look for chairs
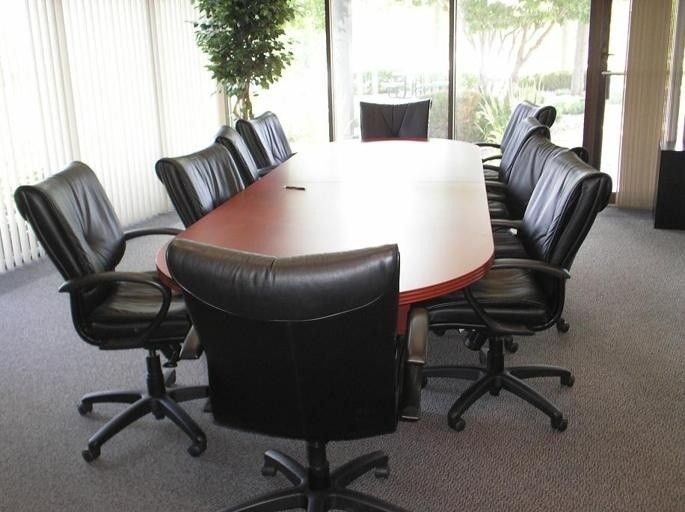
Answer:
[358,99,431,142]
[424,98,590,352]
[13,160,214,462]
[155,144,244,228]
[213,125,279,189]
[13,96,613,512]
[166,235,409,512]
[235,110,299,170]
[423,151,612,433]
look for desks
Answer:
[651,144,685,230]
[154,138,495,389]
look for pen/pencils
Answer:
[286,186,305,190]
